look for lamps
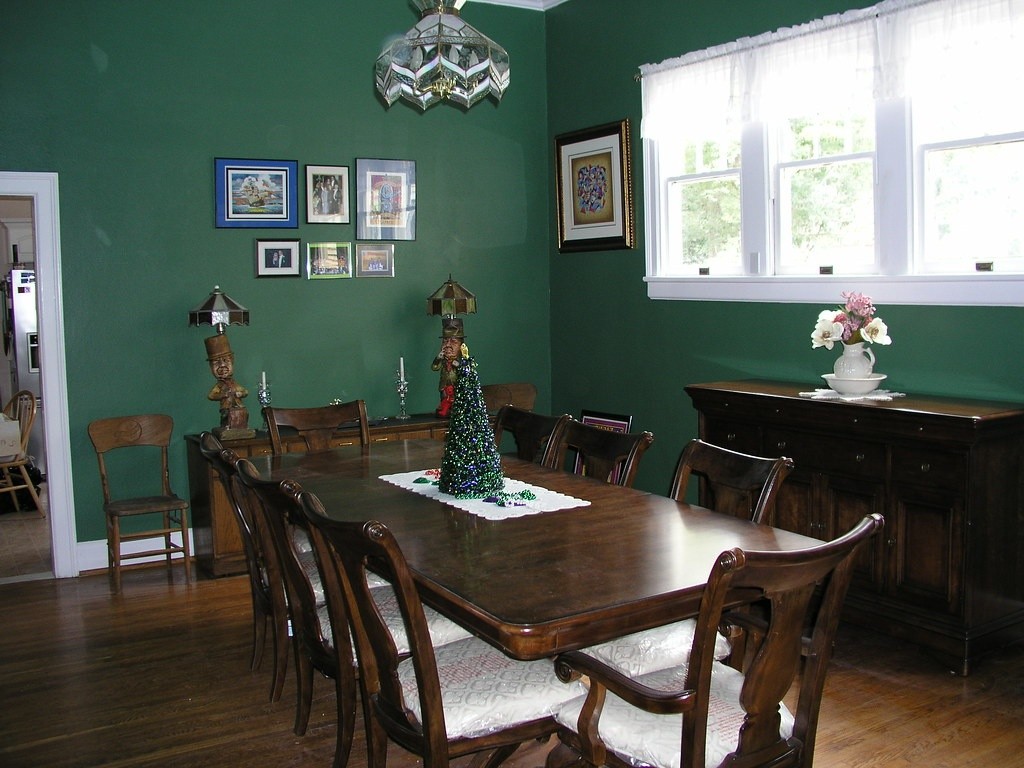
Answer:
[427,272,478,416]
[372,0,511,111]
[187,285,258,441]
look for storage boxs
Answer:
[0,418,22,457]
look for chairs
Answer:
[545,416,655,487]
[493,404,568,466]
[197,427,392,705]
[545,511,886,768]
[262,395,371,552]
[87,414,192,591]
[0,390,47,518]
[278,477,589,768]
[481,383,537,416]
[233,458,474,768]
[576,437,795,679]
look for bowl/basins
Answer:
[820,373,888,397]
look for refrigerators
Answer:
[10,270,46,474]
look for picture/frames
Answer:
[575,410,633,484]
[356,244,396,278]
[554,119,635,253]
[355,157,417,241]
[307,242,353,280]
[305,164,350,225]
[255,238,302,279]
[214,158,299,229]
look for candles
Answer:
[262,371,267,398]
[399,357,404,383]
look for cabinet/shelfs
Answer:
[181,411,497,582]
[682,380,1024,678]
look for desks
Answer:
[247,440,828,768]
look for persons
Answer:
[432,318,467,408]
[204,334,248,427]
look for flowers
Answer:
[808,291,892,349]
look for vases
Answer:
[833,339,875,378]
